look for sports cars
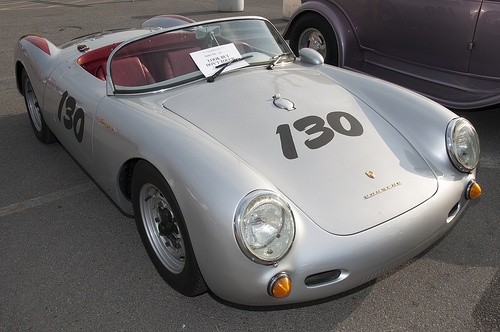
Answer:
[13,15,482,309]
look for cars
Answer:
[281,0,500,111]
